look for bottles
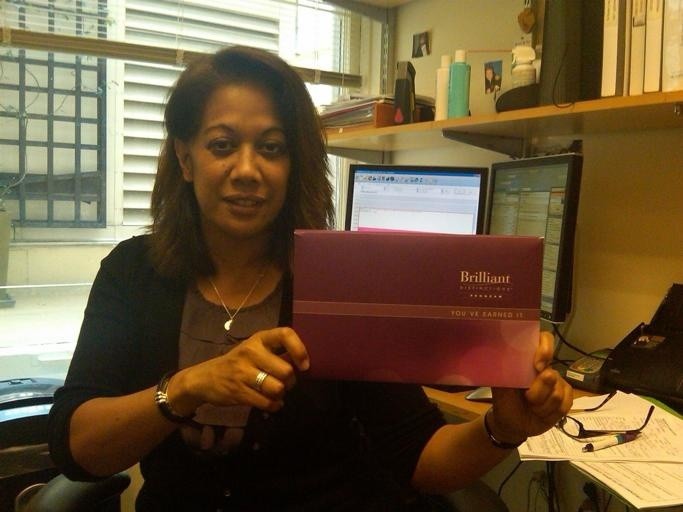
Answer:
[434,54,451,120]
[447,49,470,118]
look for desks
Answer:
[420,376,682,511]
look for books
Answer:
[534,1,683,107]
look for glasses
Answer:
[550,391,655,440]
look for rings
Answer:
[253,371,267,391]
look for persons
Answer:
[414,33,429,56]
[46,46,573,512]
[484,62,499,93]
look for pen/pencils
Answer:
[582,432,637,453]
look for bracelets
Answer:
[483,408,523,448]
[154,369,186,425]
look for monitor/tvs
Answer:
[483,151,584,324]
[344,164,490,235]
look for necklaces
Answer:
[208,265,263,332]
